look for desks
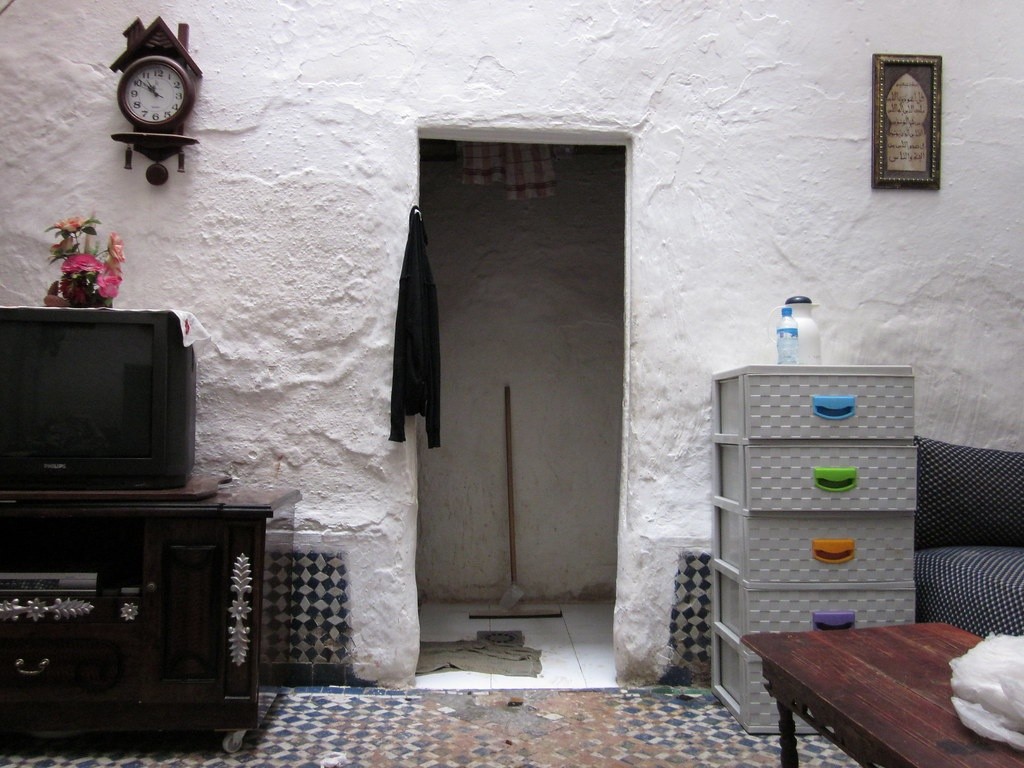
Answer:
[742,623,1024,768]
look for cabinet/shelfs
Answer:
[711,366,916,735]
[0,488,301,750]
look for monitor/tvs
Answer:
[0,307,197,490]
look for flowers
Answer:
[44,216,125,307]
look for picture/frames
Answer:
[872,54,942,190]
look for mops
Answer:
[468,384,564,619]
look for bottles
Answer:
[777,307,801,365]
[786,294,822,368]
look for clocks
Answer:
[111,16,203,187]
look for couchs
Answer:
[916,437,1024,639]
